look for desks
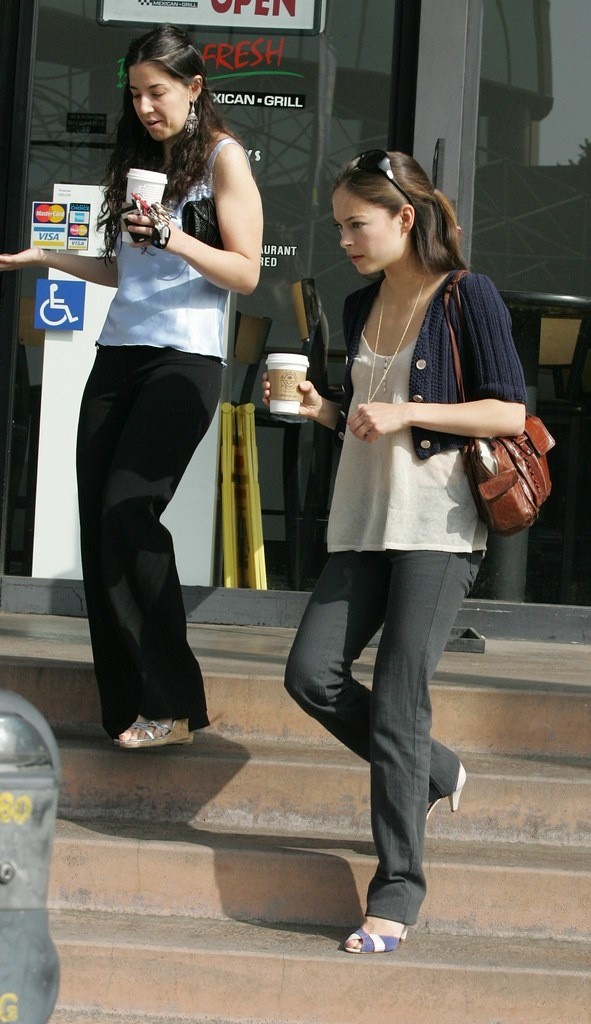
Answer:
[483,289,591,604]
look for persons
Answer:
[261,151,527,954]
[0,23,265,747]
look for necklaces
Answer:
[368,274,426,403]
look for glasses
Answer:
[354,149,414,208]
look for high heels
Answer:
[344,912,409,955]
[428,759,468,820]
[112,712,192,750]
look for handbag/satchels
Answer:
[441,268,557,535]
[182,196,223,249]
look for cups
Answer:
[266,353,309,415]
[122,168,168,243]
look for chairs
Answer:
[5,302,45,577]
[233,277,351,588]
[527,316,591,604]
